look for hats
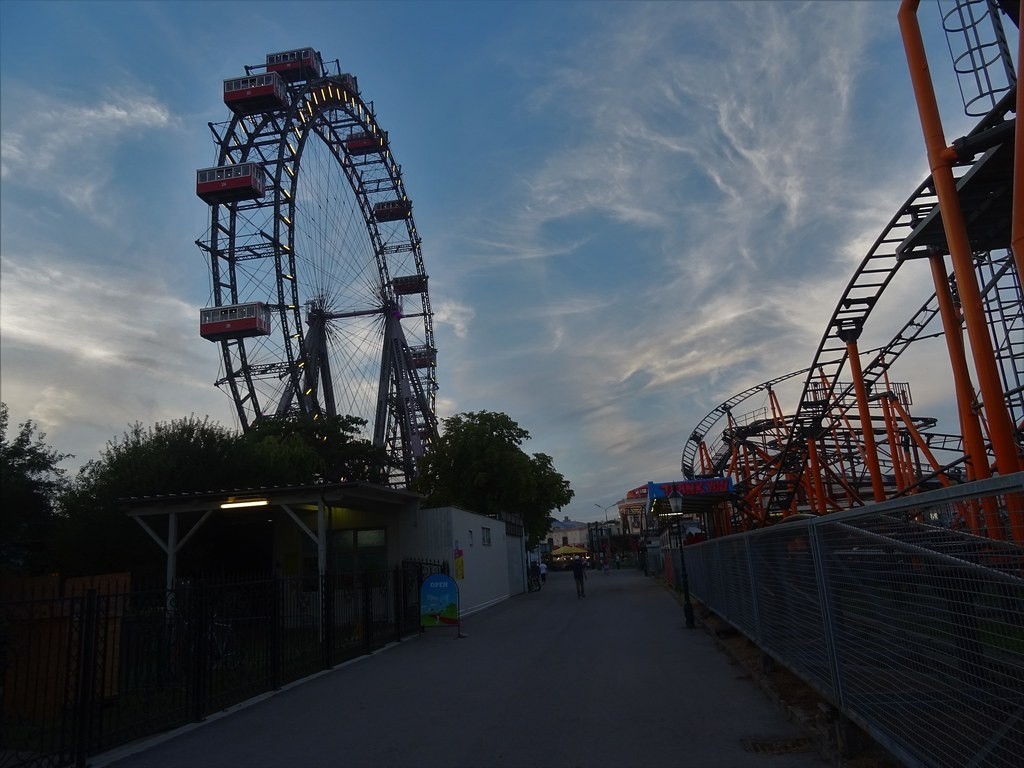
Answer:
[575,555,580,561]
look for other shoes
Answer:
[542,582,544,585]
[577,595,580,598]
[581,594,585,597]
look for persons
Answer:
[599,552,620,576]
[556,553,591,562]
[574,555,585,597]
[529,560,542,592]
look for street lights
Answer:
[667,480,695,629]
[595,502,618,521]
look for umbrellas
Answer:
[551,545,588,555]
